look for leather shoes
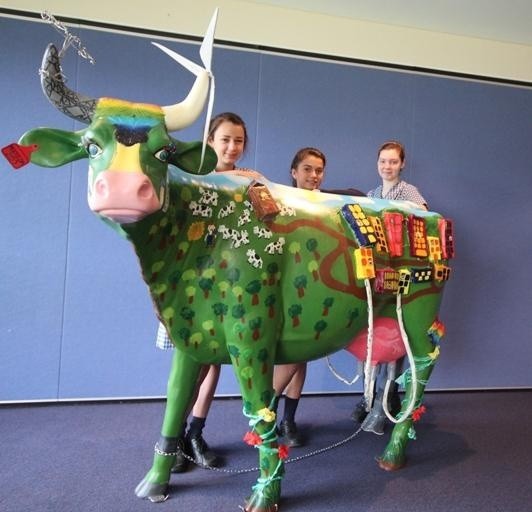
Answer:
[190,436,218,469]
[389,392,401,417]
[280,420,302,448]
[350,399,370,424]
[170,440,188,473]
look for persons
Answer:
[156,112,268,472]
[271,147,366,448]
[349,141,427,421]
